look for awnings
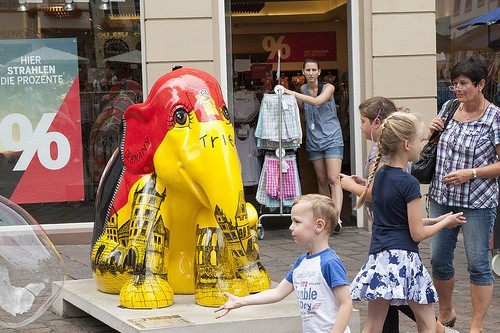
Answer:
[456,8,500,30]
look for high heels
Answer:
[440,310,456,327]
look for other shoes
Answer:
[333,220,342,233]
[444,325,460,333]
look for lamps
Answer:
[64,0,89,11]
[17,0,43,12]
[98,0,125,10]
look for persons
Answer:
[93,73,136,102]
[338,96,467,333]
[429,57,500,333]
[282,59,344,233]
[214,193,353,333]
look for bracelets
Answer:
[472,168,477,179]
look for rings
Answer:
[432,123,435,126]
[453,181,455,184]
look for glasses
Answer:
[449,82,476,91]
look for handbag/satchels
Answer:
[410,98,462,184]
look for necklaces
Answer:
[311,105,316,130]
[460,97,485,120]
